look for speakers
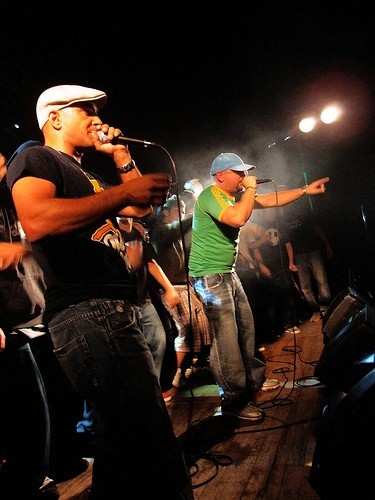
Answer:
[319,286,369,339]
[313,303,374,388]
[316,352,375,436]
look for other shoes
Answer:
[162,393,172,402]
[172,371,182,388]
[286,326,300,333]
[185,358,200,378]
[309,312,321,323]
[221,403,264,421]
[259,379,280,390]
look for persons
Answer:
[115,178,213,402]
[6,85,195,500]
[188,153,330,421]
[235,185,330,352]
[0,141,94,500]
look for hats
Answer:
[36,85,107,129]
[210,152,255,176]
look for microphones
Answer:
[95,130,155,146]
[237,177,270,191]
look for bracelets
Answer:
[302,184,311,197]
[115,159,135,173]
[245,186,257,194]
